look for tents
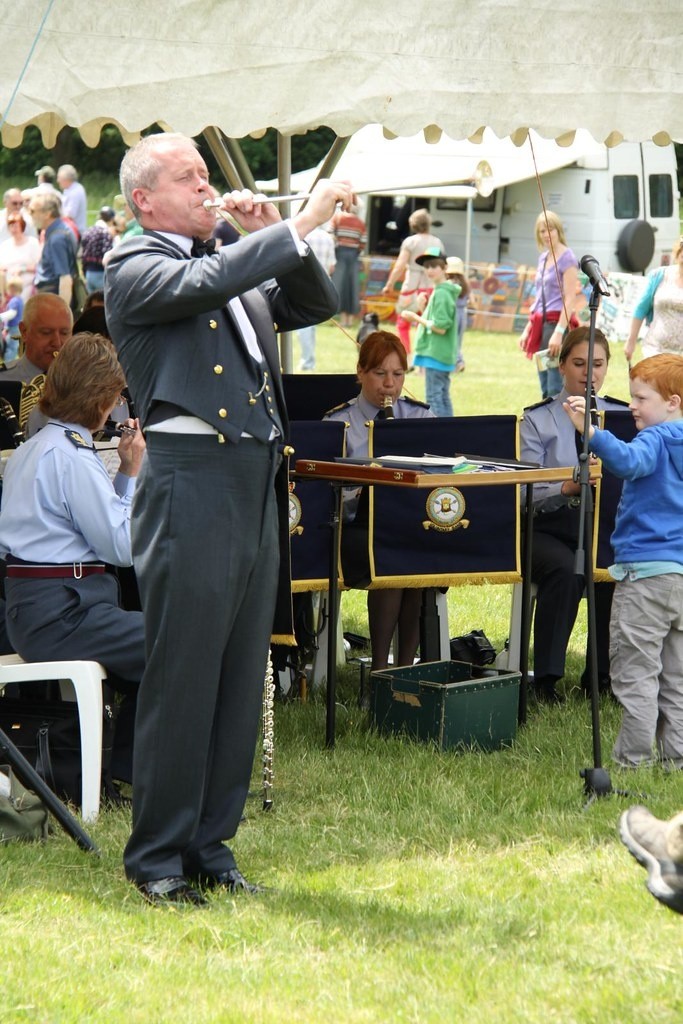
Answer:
[0,0,683,169]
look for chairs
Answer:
[0,336,650,853]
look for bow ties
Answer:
[191,234,220,259]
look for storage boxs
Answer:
[369,660,522,752]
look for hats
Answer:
[415,247,447,264]
[446,257,463,274]
[34,166,56,178]
[101,206,115,216]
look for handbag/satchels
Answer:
[450,629,497,666]
[72,277,90,321]
[0,764,56,842]
[534,345,565,371]
[0,693,119,804]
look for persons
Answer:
[625,238,683,366]
[562,353,683,773]
[400,247,462,416]
[0,332,146,807]
[382,209,444,374]
[323,331,437,697]
[288,192,367,372]
[0,164,153,451]
[518,325,633,701]
[445,257,469,372]
[103,132,357,912]
[621,806,683,916]
[519,209,579,402]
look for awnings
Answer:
[255,122,590,294]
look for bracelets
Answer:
[554,326,565,334]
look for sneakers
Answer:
[195,866,280,897]
[136,874,209,905]
[619,805,683,912]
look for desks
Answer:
[294,457,614,794]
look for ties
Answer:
[377,410,387,420]
[575,428,597,502]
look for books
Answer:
[334,450,548,473]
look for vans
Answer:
[255,124,679,275]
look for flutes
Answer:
[0,397,25,447]
[585,381,598,489]
[380,393,395,419]
[105,420,136,436]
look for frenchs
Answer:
[18,350,58,432]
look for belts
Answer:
[4,567,105,578]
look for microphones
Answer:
[580,255,611,297]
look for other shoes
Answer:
[457,361,464,371]
[535,683,563,702]
[575,690,604,702]
